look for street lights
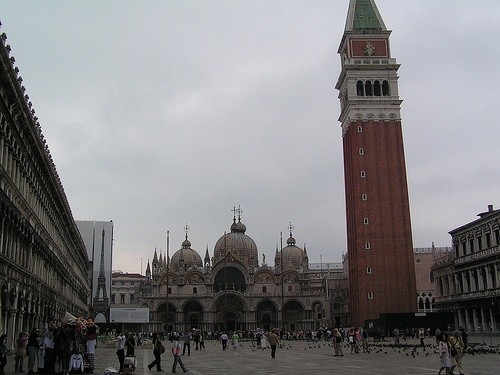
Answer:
[166,230,170,331]
[90,220,96,306]
[279,232,285,330]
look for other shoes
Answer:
[14,369,19,373]
[157,368,163,372]
[19,370,25,373]
[28,370,36,374]
[438,372,440,375]
[184,370,188,373]
[446,374,449,375]
[460,373,464,375]
[335,354,339,356]
[339,355,344,356]
[148,365,152,371]
[173,371,177,373]
[450,372,455,375]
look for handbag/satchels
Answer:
[154,342,163,357]
[335,333,342,343]
[349,333,354,343]
[25,344,30,356]
[451,350,458,357]
[171,342,181,354]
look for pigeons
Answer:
[247,342,293,351]
[205,340,244,350]
[340,340,351,348]
[468,343,497,356]
[304,341,334,351]
[358,343,433,358]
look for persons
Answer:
[0,317,474,375]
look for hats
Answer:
[31,328,40,333]
[86,319,94,325]
[127,332,134,338]
[454,329,459,335]
